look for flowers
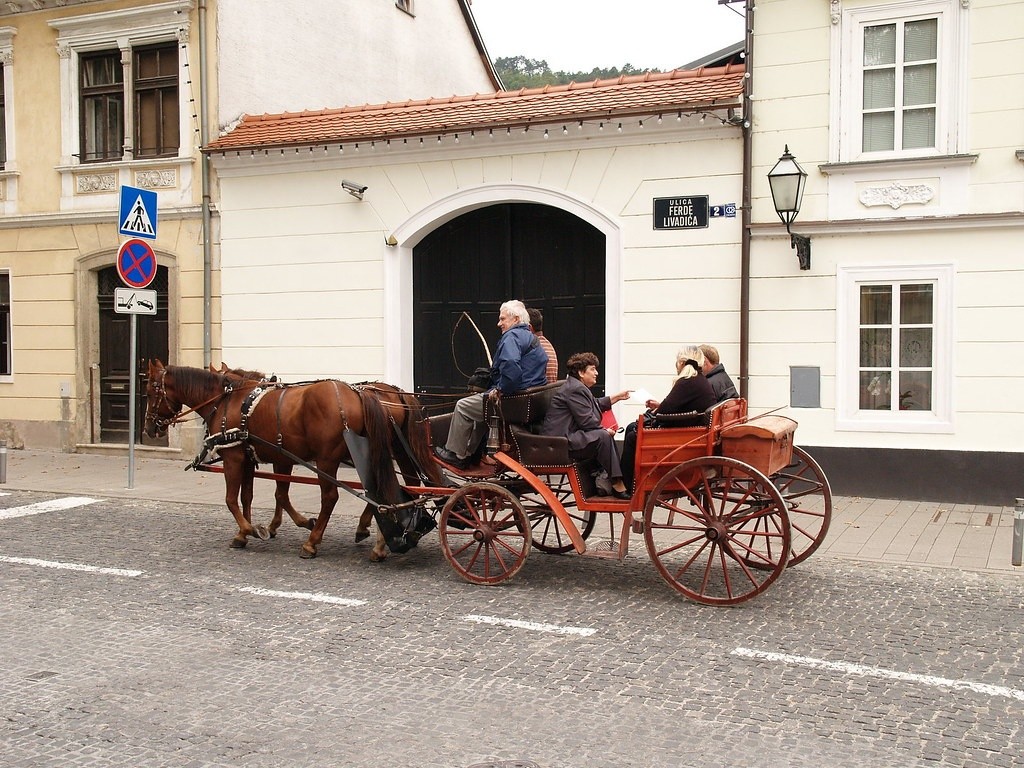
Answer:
[867,373,924,409]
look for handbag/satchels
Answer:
[634,409,661,434]
[591,468,613,496]
[466,368,494,395]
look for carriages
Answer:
[141,356,834,607]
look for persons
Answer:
[434,300,548,469]
[619,345,717,493]
[700,345,739,402]
[527,309,558,384]
[866,373,890,408]
[542,353,635,500]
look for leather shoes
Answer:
[470,454,480,466]
[612,485,632,500]
[436,447,471,470]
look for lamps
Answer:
[767,144,811,270]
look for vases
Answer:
[900,406,907,410]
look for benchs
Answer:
[635,397,747,490]
[482,379,593,475]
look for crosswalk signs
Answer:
[118,183,159,241]
